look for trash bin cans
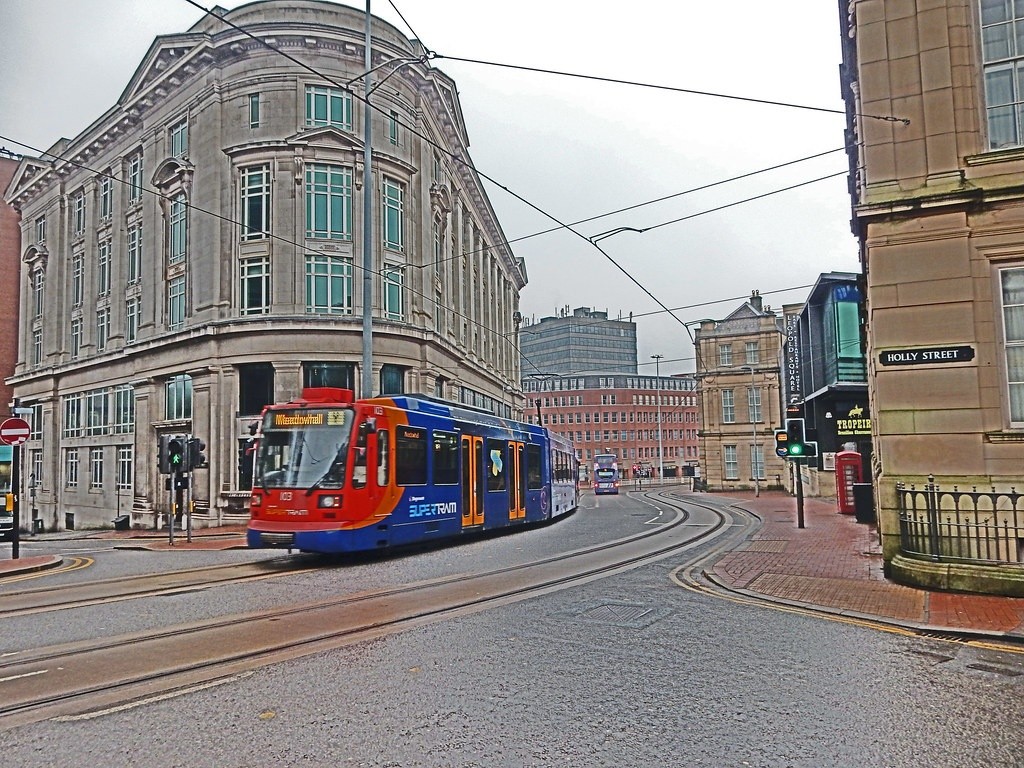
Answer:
[852,482,875,522]
[693,476,701,489]
[34,518,44,533]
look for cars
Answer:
[0,497,13,542]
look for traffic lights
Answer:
[786,417,819,458]
[190,438,206,466]
[166,435,188,471]
[5,494,13,511]
[158,431,170,475]
[238,441,252,476]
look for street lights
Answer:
[650,354,665,485]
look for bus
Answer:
[246,371,582,557]
[593,454,620,495]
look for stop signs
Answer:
[0,419,32,446]
